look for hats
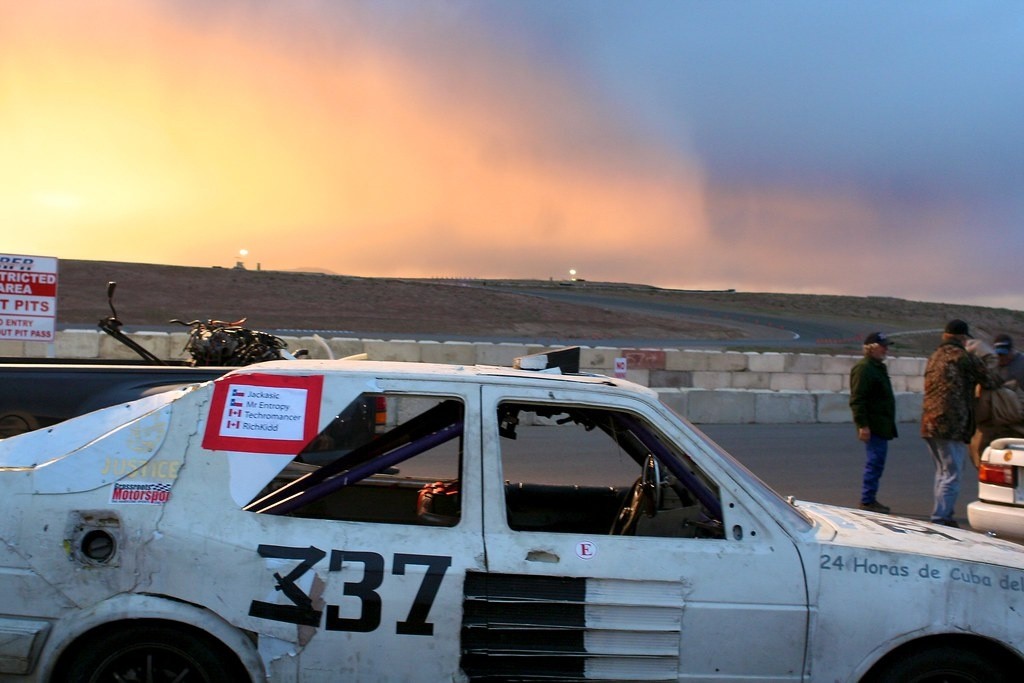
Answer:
[992,334,1011,354]
[945,319,975,340]
[864,332,895,346]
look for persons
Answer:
[921,319,1024,527]
[850,332,898,513]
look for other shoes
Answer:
[931,519,959,528]
[857,500,890,514]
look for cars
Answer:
[966,438,1024,542]
[1,347,1024,683]
